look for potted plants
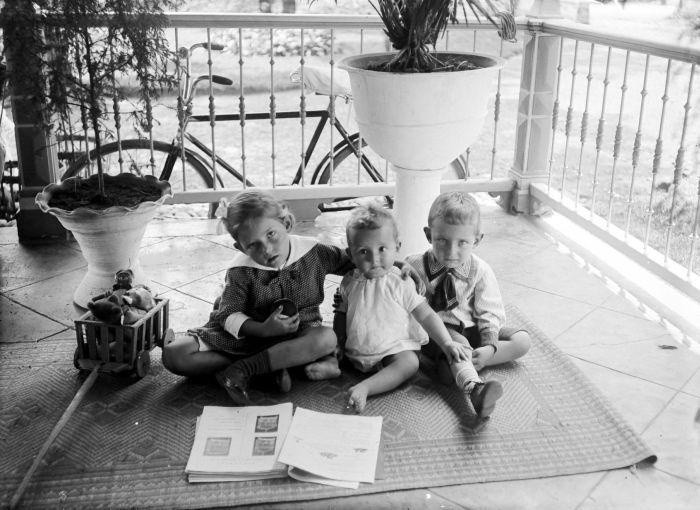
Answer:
[337,0,518,265]
[0,0,192,312]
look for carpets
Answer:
[0,297,656,509]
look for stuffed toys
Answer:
[86,270,156,326]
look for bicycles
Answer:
[56,39,474,217]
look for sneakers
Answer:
[470,380,503,418]
[215,362,291,406]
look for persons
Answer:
[304,201,473,413]
[162,189,427,405]
[331,190,531,417]
